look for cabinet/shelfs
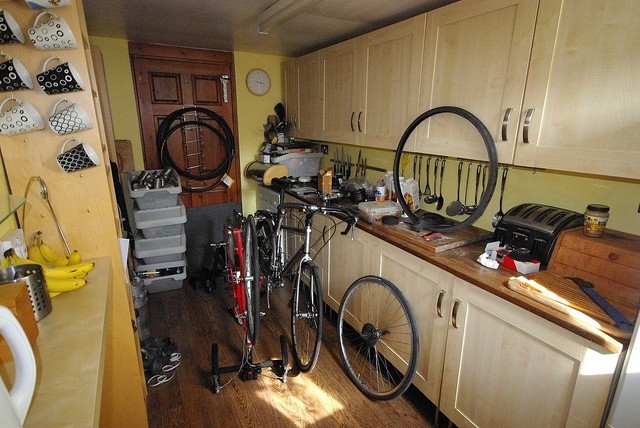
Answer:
[372,229,631,428]
[322,211,371,344]
[427,0,640,184]
[319,11,427,155]
[0,0,152,427]
[280,49,319,140]
[283,188,323,300]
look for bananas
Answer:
[1,247,94,299]
[28,230,81,266]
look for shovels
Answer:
[424,158,431,195]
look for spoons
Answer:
[424,158,440,203]
[491,168,508,228]
[445,162,463,216]
[465,164,481,214]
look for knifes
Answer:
[333,145,352,180]
[354,149,361,176]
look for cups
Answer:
[0,8,26,44]
[46,98,94,136]
[27,10,79,50]
[25,0,72,10]
[35,56,87,95]
[0,96,46,137]
[0,52,35,91]
[56,138,101,175]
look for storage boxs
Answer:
[269,150,323,176]
[127,167,182,209]
[133,253,188,294]
[132,232,186,264]
[132,196,188,238]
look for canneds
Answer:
[583,204,610,239]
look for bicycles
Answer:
[254,202,359,374]
[203,207,291,392]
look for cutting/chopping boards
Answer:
[371,208,494,253]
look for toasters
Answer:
[491,201,586,269]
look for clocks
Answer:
[246,69,271,96]
